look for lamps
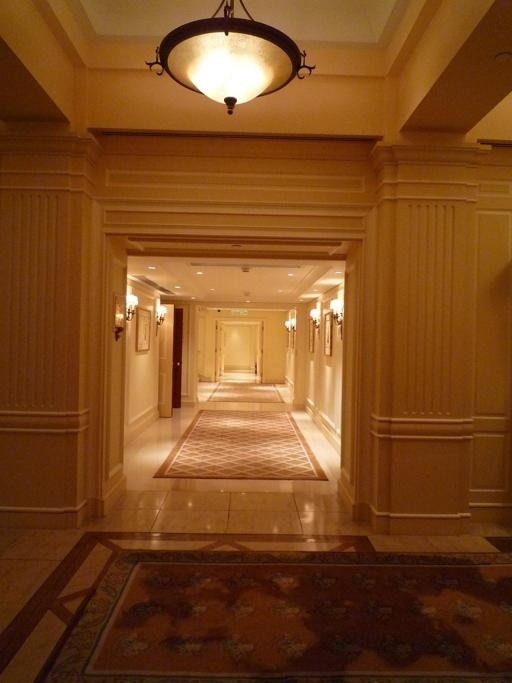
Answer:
[145,0,313,112]
[286,319,296,332]
[126,293,138,320]
[310,309,320,333]
[156,305,167,335]
[113,295,124,340]
[330,298,342,338]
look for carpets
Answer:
[207,382,284,403]
[30,549,511,682]
[152,408,329,481]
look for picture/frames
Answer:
[136,307,151,352]
[324,311,332,356]
[308,318,314,352]
